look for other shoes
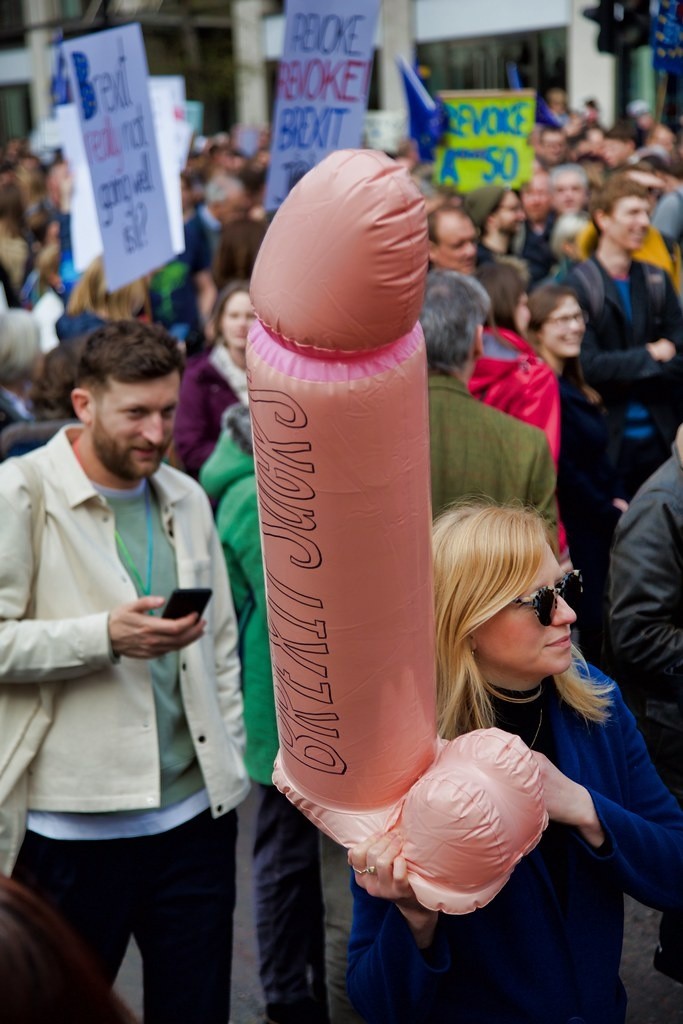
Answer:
[264,1002,332,1024]
[652,944,683,984]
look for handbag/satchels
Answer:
[0,456,59,878]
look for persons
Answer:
[2,85,682,671]
[345,494,683,1024]
[1,869,142,1023]
[199,402,330,1023]
[0,320,251,1024]
[416,268,559,562]
[600,422,683,811]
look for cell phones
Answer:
[161,588,213,623]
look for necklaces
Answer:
[530,707,543,751]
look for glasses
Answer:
[509,570,583,627]
[543,311,589,327]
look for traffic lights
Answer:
[582,0,613,57]
[615,2,651,53]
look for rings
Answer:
[352,865,366,874]
[365,864,376,875]
